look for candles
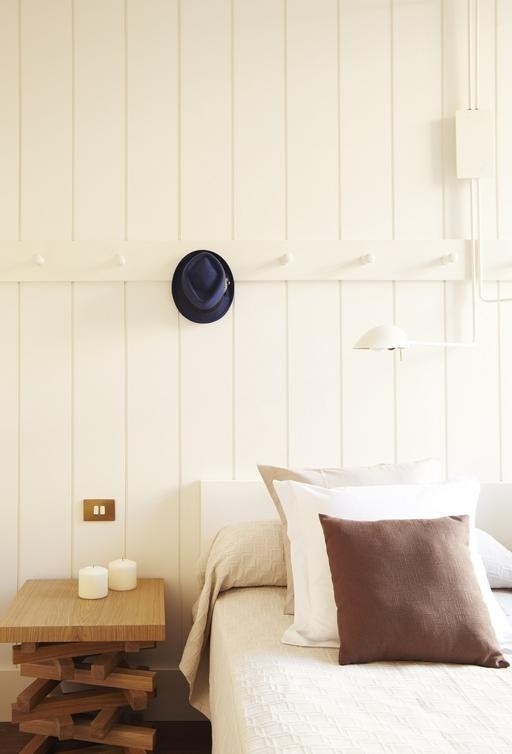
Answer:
[108,557,137,591]
[78,564,108,600]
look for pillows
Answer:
[178,518,512,721]
[256,457,512,668]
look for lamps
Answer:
[353,0,490,351]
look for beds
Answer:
[200,481,512,754]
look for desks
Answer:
[0,578,166,754]
